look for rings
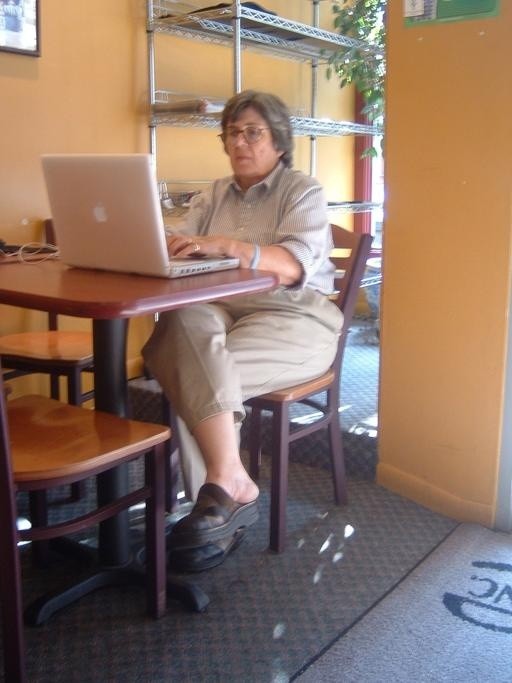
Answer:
[184,237,195,245]
[190,242,202,253]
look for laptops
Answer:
[39,153,240,279]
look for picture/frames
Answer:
[0,0,39,56]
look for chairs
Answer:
[0,217,97,501]
[0,375,173,681]
[159,222,374,556]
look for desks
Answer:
[0,247,280,628]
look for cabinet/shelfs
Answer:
[144,0,386,298]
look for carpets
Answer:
[286,519,512,682]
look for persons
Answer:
[137,87,349,579]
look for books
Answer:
[149,99,226,115]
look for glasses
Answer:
[217,127,271,145]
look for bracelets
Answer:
[248,242,262,271]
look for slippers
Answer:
[175,483,259,543]
[171,528,246,571]
